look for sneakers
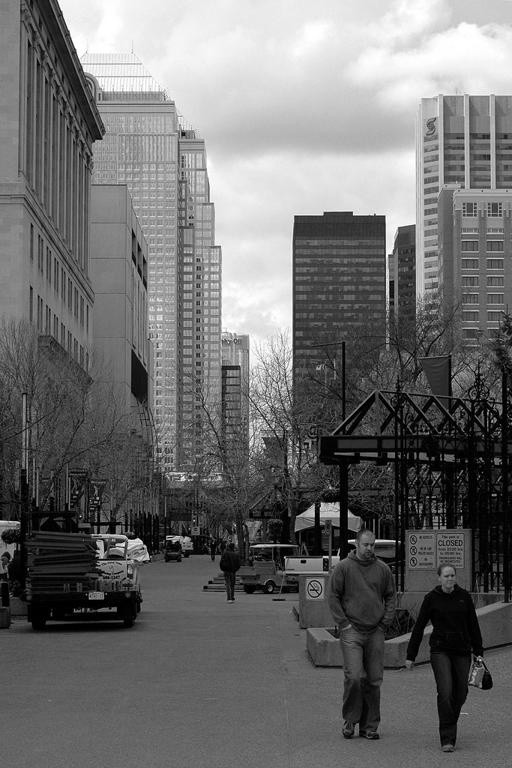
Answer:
[343,720,379,739]
[442,744,454,752]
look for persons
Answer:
[405,564,483,752]
[220,544,240,602]
[0,551,20,607]
[210,539,235,560]
[327,528,397,740]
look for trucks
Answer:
[164,535,193,563]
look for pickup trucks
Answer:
[284,540,407,590]
[26,512,143,628]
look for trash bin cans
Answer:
[0,582,11,629]
[150,554,153,563]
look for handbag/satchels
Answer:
[468,657,493,689]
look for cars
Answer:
[239,544,300,594]
